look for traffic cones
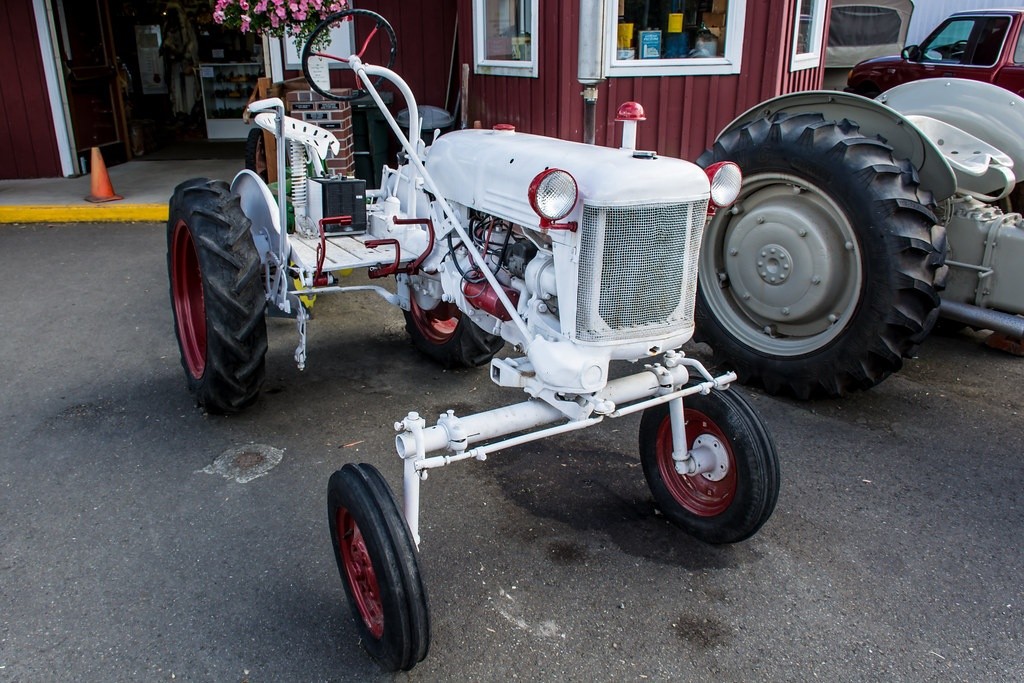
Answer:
[83,146,125,204]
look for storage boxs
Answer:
[617,49,635,60]
[696,0,727,52]
[617,23,634,49]
[637,27,662,59]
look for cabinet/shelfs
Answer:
[198,62,268,139]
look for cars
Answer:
[841,9,1024,101]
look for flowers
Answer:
[213,0,352,61]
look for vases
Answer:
[511,36,531,61]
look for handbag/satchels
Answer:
[163,46,184,64]
[182,56,195,74]
